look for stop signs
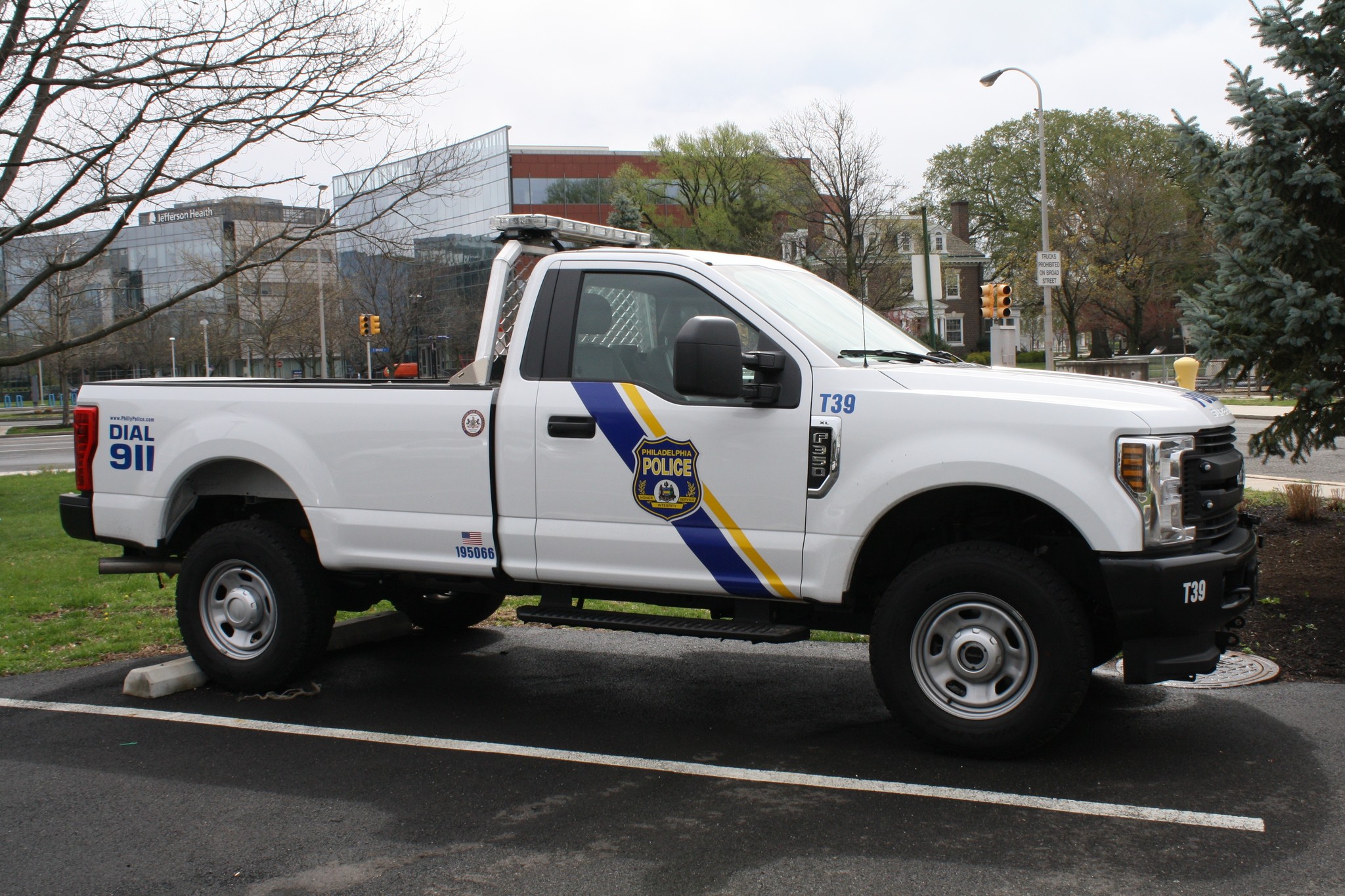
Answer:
[276,361,284,369]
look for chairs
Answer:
[593,292,633,380]
[650,298,700,396]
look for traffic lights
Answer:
[996,285,1011,317]
[360,315,369,335]
[370,316,381,334]
[978,285,995,318]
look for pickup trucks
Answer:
[58,214,1270,761]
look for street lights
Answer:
[170,338,177,377]
[33,345,45,407]
[410,294,423,378]
[981,68,1056,372]
[316,184,329,379]
[199,319,210,377]
